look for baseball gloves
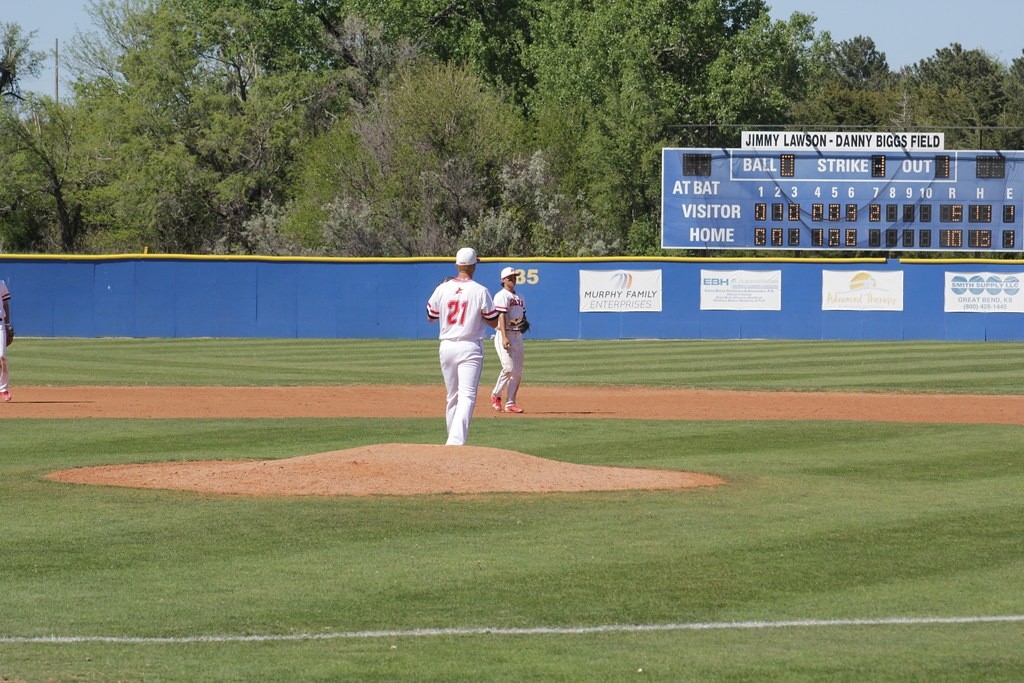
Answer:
[5,324,17,347]
[435,276,454,287]
[510,317,532,333]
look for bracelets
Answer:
[5,324,11,326]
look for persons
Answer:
[0,280,15,402]
[426,248,499,445]
[490,267,530,413]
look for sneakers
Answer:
[504,401,524,413]
[491,395,503,412]
[0,391,12,401]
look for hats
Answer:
[501,267,521,278]
[456,247,480,265]
